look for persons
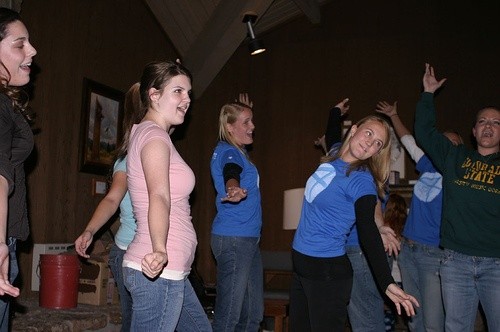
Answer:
[74,58,264,332]
[287,63,500,332]
[0,7,38,332]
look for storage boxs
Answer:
[78,260,119,305]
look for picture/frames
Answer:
[78,77,126,175]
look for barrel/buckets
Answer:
[36,253,83,311]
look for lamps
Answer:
[283,189,306,229]
[242,14,266,56]
